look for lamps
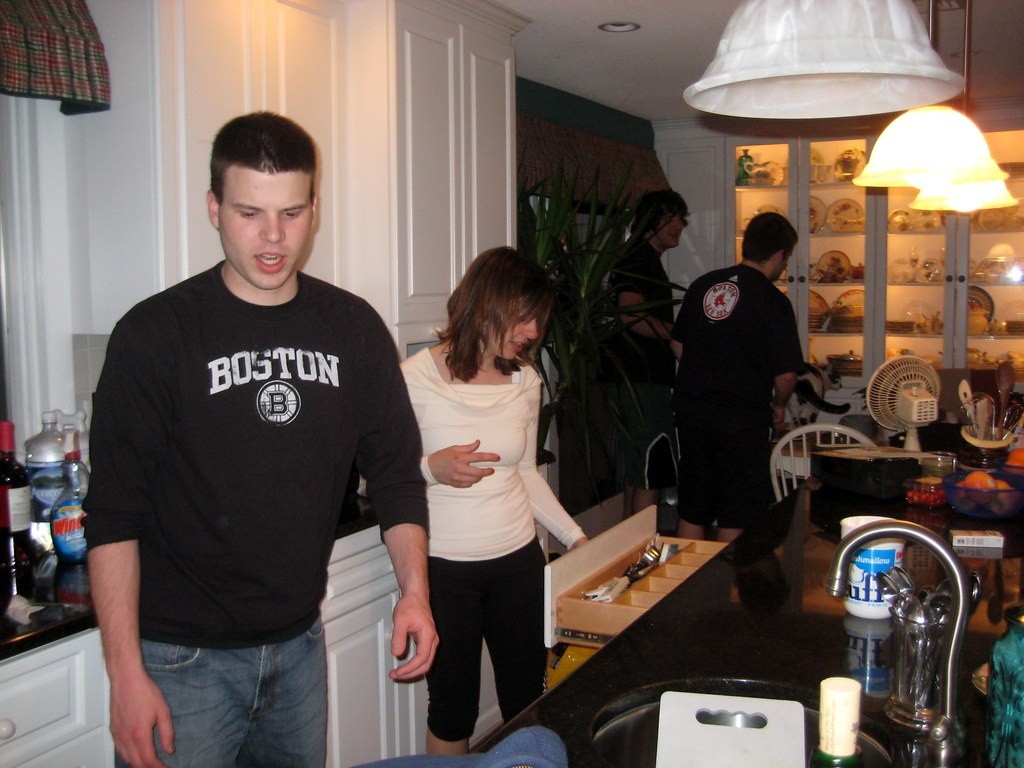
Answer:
[852,0,1010,188]
[909,0,1019,212]
[683,0,964,119]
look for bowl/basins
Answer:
[942,469,1024,517]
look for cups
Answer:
[842,611,894,699]
[882,612,953,733]
[837,514,908,620]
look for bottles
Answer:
[23,411,68,552]
[808,743,867,768]
[0,420,32,576]
[737,149,754,186]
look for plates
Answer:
[761,161,785,186]
[807,147,867,334]
[886,201,1023,338]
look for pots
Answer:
[827,350,862,382]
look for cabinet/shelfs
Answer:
[0,1,519,768]
[725,129,1024,398]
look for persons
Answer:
[399,246,586,754]
[81,113,438,768]
[607,189,690,510]
[669,213,802,543]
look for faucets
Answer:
[825,519,971,768]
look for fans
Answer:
[866,354,941,451]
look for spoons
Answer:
[874,563,981,705]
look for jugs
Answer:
[740,161,776,186]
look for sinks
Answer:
[592,697,893,768]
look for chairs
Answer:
[770,422,880,494]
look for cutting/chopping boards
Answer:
[654,690,807,768]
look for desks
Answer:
[472,465,1024,768]
